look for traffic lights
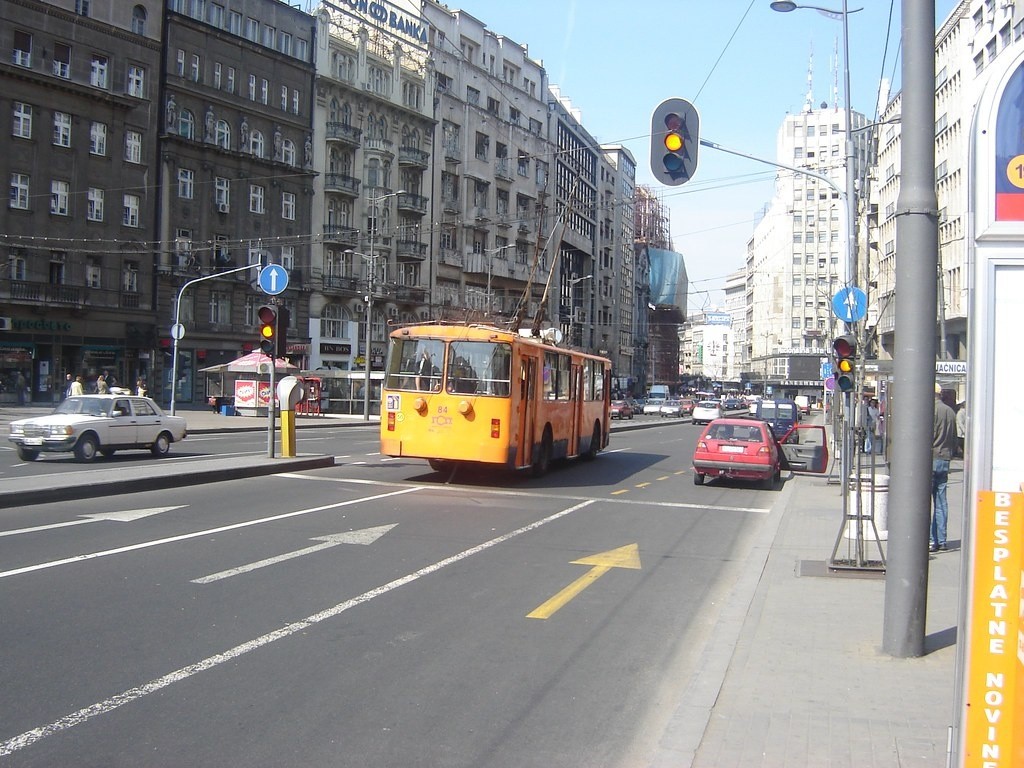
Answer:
[648,97,701,187]
[832,334,857,394]
[258,305,277,355]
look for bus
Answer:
[378,176,613,481]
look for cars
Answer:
[6,393,188,464]
[607,383,812,445]
[691,417,829,490]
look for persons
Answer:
[103,370,112,386]
[954,400,965,449]
[413,346,440,391]
[96,375,109,394]
[929,382,958,551]
[860,395,880,453]
[64,373,84,414]
[135,377,149,397]
[17,371,25,405]
[614,388,624,400]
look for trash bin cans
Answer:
[826,409,832,425]
[843,472,889,540]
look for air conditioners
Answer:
[0,316,12,331]
[354,304,364,313]
[390,308,399,317]
[217,203,229,214]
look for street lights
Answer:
[344,189,410,422]
[644,344,670,385]
[768,0,902,476]
[554,274,593,346]
[467,242,517,320]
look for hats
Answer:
[934,382,941,393]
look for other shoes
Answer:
[935,541,948,550]
[928,542,935,552]
